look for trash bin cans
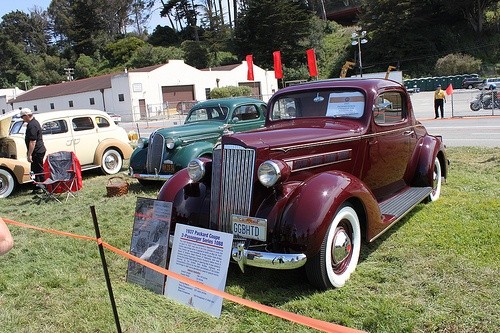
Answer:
[412,78,420,91]
[428,77,433,91]
[440,76,448,90]
[420,78,428,91]
[449,76,455,89]
[404,79,412,92]
[433,77,439,91]
[455,76,461,89]
[461,75,469,81]
[469,74,478,79]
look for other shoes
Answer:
[28,189,44,195]
[32,191,45,199]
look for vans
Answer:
[483,78,500,88]
[176,100,199,114]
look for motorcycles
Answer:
[470,91,500,111]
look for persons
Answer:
[434,84,447,119]
[0,217,14,256]
[20,108,47,195]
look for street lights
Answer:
[351,30,368,78]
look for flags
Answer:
[246,55,254,80]
[446,84,454,94]
[306,50,317,76]
[273,51,283,79]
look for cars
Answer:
[478,79,496,90]
[128,97,281,188]
[105,112,121,125]
[0,110,133,199]
[157,78,450,290]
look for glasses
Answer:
[20,114,30,119]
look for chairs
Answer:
[29,151,82,205]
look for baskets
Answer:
[105,176,129,197]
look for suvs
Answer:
[462,77,482,89]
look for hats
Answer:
[19,108,33,117]
